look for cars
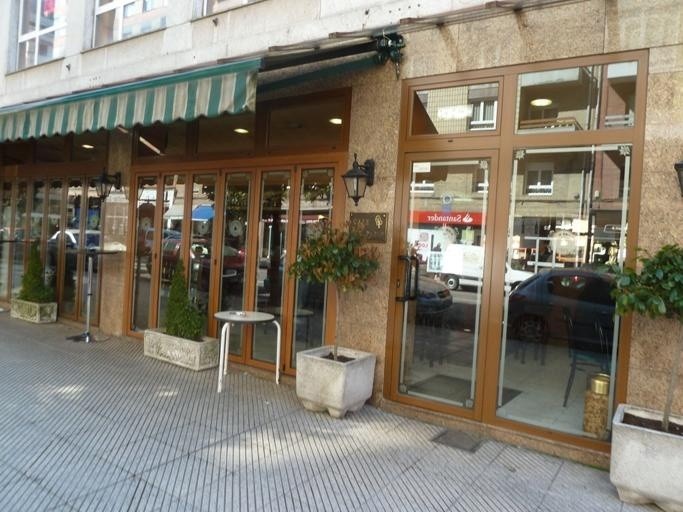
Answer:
[145,234,246,296]
[0,227,41,257]
[42,228,126,274]
[268,246,455,326]
[508,267,620,354]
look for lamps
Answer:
[339,151,376,208]
[91,164,122,204]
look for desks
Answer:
[61,247,121,343]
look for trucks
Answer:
[438,244,534,296]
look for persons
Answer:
[192,247,203,305]
[434,243,441,280]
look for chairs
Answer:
[508,300,553,367]
[410,303,453,367]
[554,305,608,409]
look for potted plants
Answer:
[141,257,221,373]
[6,237,60,325]
[603,240,682,511]
[288,221,382,418]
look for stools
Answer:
[210,308,283,397]
[265,307,313,350]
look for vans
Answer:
[21,212,67,237]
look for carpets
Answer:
[411,374,524,414]
